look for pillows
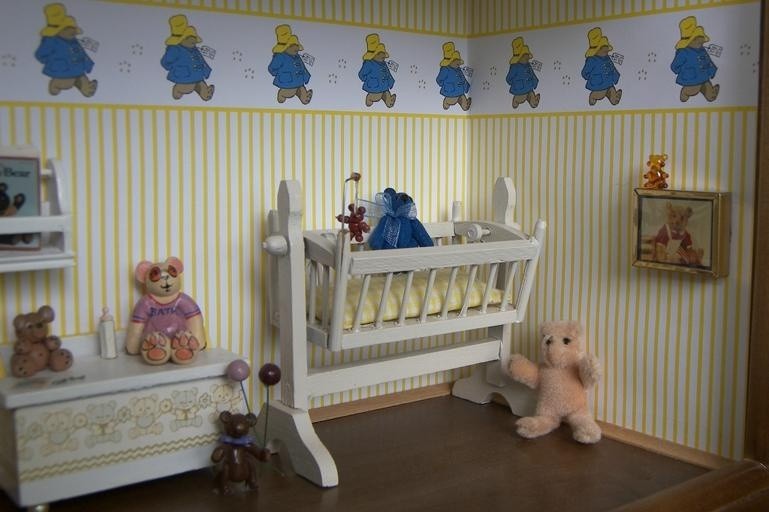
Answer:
[306,268,512,329]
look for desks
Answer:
[0,328,247,512]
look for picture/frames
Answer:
[630,187,731,279]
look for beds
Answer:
[256,176,547,487]
